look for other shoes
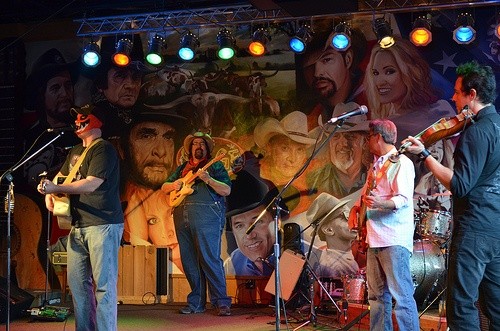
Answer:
[217,305,231,316]
[180,306,205,313]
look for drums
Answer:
[414,208,453,239]
[407,237,447,292]
[341,275,366,303]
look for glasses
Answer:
[365,133,379,142]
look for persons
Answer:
[401,66,500,331]
[35,31,452,279]
[39,102,125,331]
[162,132,232,316]
[350,121,421,331]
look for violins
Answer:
[388,108,477,164]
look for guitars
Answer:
[169,152,227,207]
[358,143,377,254]
[40,179,73,229]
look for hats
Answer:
[183,131,215,154]
[70,102,107,124]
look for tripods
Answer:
[266,212,343,331]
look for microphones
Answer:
[328,105,368,124]
[47,126,76,133]
[261,258,275,271]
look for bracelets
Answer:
[204,178,212,185]
[418,149,430,163]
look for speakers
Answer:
[0,276,35,324]
